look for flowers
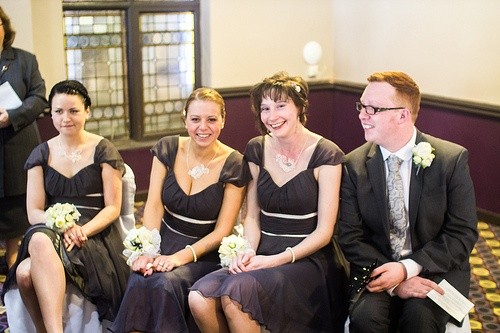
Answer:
[411,138,435,174]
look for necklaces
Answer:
[56,132,89,162]
[267,137,309,171]
[187,140,221,180]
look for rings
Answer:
[158,264,163,267]
[69,234,73,237]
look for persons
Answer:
[107,88,254,333]
[0,6,47,271]
[334,71,479,333]
[189,72,345,333]
[15,80,130,333]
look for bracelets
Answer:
[286,247,295,264]
[185,244,197,263]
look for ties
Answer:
[386,153,408,262]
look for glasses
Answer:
[344,257,377,294]
[355,100,406,115]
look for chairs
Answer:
[3,162,137,333]
